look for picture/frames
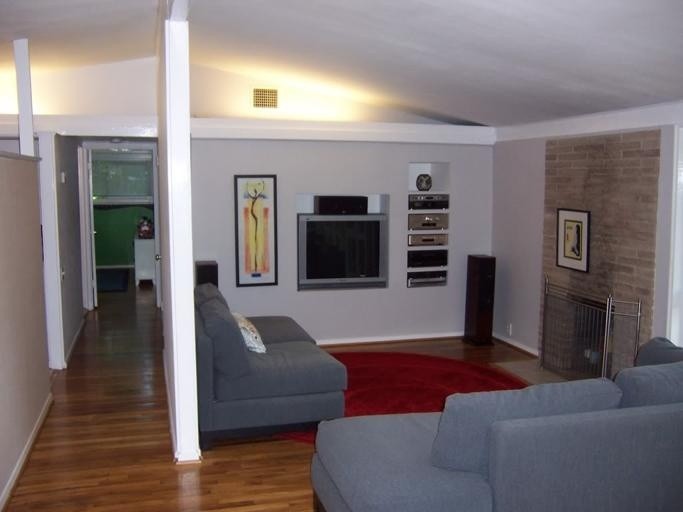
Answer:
[555,207,591,274]
[233,173,279,288]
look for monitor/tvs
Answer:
[297,213,389,291]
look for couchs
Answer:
[310,336,683,512]
[195,315,350,452]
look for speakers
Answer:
[464,255,496,346]
[195,260,218,289]
[313,195,368,214]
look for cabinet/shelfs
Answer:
[133,234,155,287]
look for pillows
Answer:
[428,375,623,474]
[194,282,251,379]
[614,361,683,408]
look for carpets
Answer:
[268,351,535,444]
